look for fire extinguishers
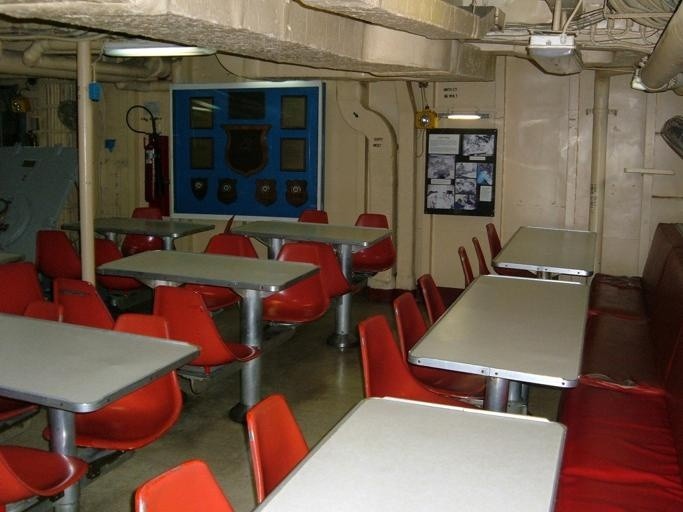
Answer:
[126,104,162,202]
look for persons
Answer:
[428,134,493,210]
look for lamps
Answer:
[102,37,214,61]
